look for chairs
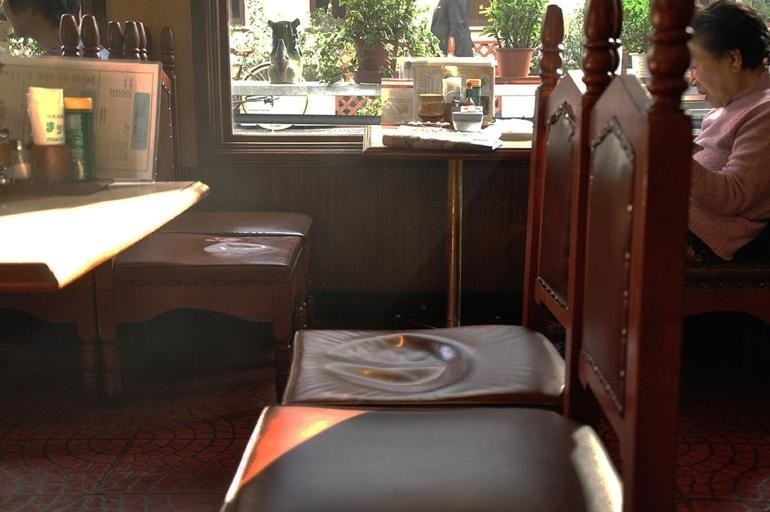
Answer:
[680,263,770,325]
[280,0,618,411]
[220,1,697,512]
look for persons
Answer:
[431,0,474,57]
[688,0,770,268]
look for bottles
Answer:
[0,127,31,184]
[466,79,481,106]
[380,76,416,124]
[65,96,94,182]
[418,94,444,123]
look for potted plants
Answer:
[294,0,446,87]
[617,0,656,79]
[476,0,549,78]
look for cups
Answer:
[33,144,64,185]
[452,111,484,133]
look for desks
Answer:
[360,123,533,329]
